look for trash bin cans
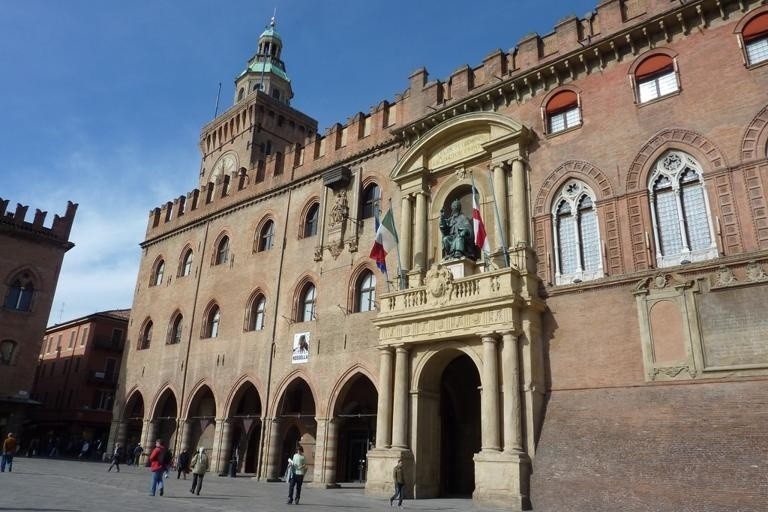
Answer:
[229,460,237,477]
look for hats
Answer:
[138,442,141,445]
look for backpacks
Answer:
[157,445,172,465]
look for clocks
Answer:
[207,149,240,201]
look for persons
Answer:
[439,201,472,259]
[390,460,405,507]
[0,432,17,472]
[28,438,209,496]
[286,446,308,505]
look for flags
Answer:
[374,204,387,273]
[369,208,399,263]
[472,185,491,253]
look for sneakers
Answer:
[286,500,292,504]
[149,493,155,496]
[190,489,194,494]
[397,505,403,508]
[160,487,163,496]
[9,470,11,472]
[196,492,200,495]
[295,501,299,504]
[1,470,5,472]
[390,497,392,506]
